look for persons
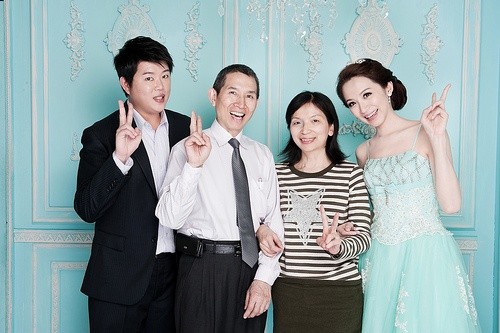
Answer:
[73,36,197,333]
[256,91,372,333]
[337,58,481,333]
[155,64,284,333]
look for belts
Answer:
[203,244,242,257]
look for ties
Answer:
[228,138,258,269]
